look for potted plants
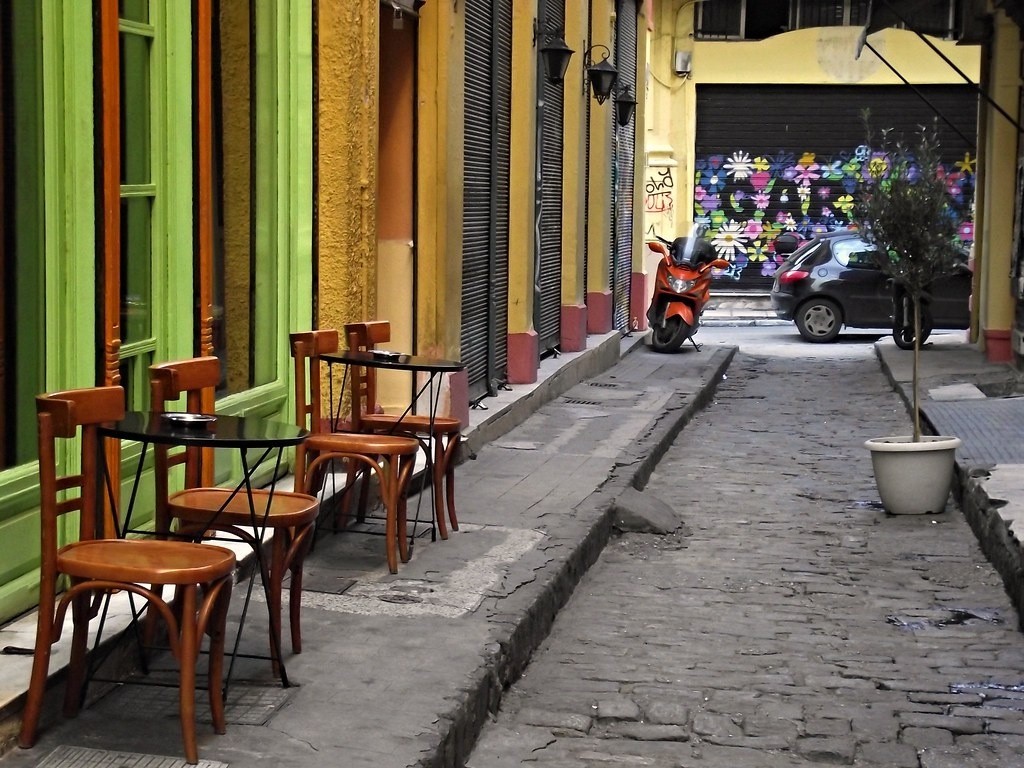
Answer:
[850,124,978,515]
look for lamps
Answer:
[583,39,619,105]
[609,78,638,128]
[532,16,576,85]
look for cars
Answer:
[768,229,973,344]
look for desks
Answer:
[310,351,465,561]
[69,412,310,711]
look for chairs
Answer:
[336,321,459,541]
[146,355,320,677]
[289,329,418,574]
[19,386,236,764]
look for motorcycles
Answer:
[647,235,731,351]
